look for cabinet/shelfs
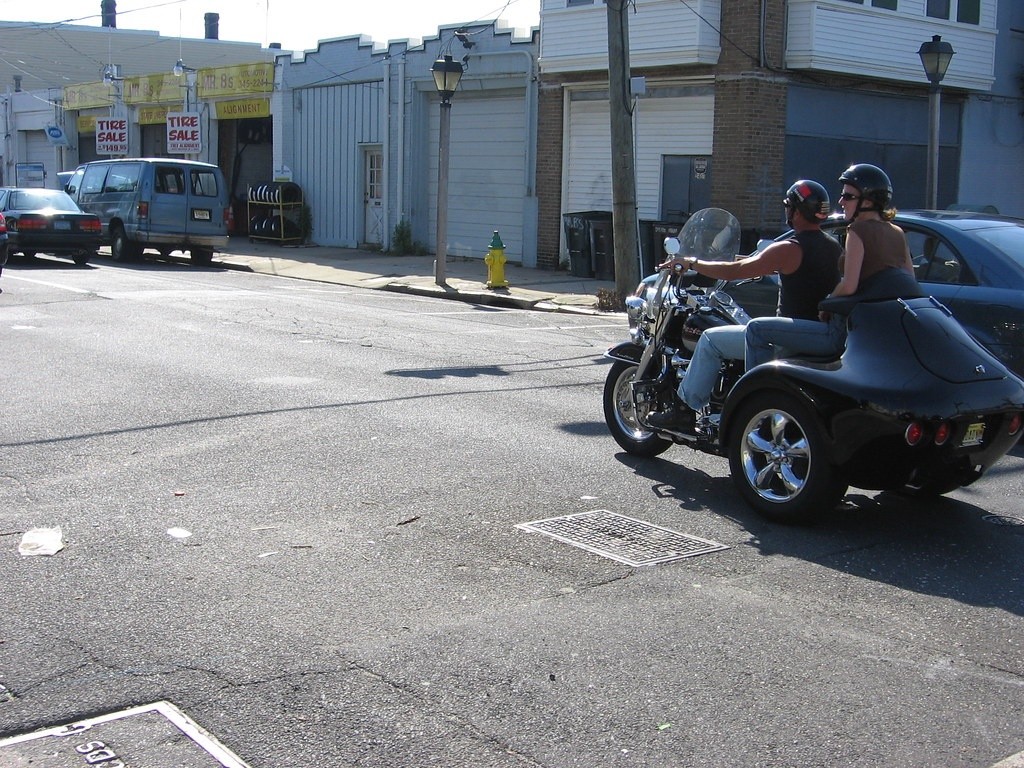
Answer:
[247,184,304,245]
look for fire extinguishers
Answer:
[228,203,235,232]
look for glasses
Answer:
[841,192,860,201]
[783,198,794,208]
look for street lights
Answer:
[429,52,465,289]
[915,34,957,197]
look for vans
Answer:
[61,155,234,267]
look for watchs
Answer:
[689,256,699,270]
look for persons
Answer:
[740,164,913,372]
[645,179,844,431]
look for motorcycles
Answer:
[602,209,1024,526]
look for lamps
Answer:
[173,59,198,76]
[104,71,124,87]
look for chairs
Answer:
[914,237,934,279]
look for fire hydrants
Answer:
[484,230,509,288]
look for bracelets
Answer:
[826,294,831,299]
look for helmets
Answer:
[838,163,893,208]
[786,179,830,223]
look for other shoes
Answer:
[646,396,696,435]
[709,414,720,426]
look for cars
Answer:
[745,209,1024,376]
[0,187,104,266]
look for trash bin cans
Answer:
[638,219,788,285]
[563,210,614,280]
